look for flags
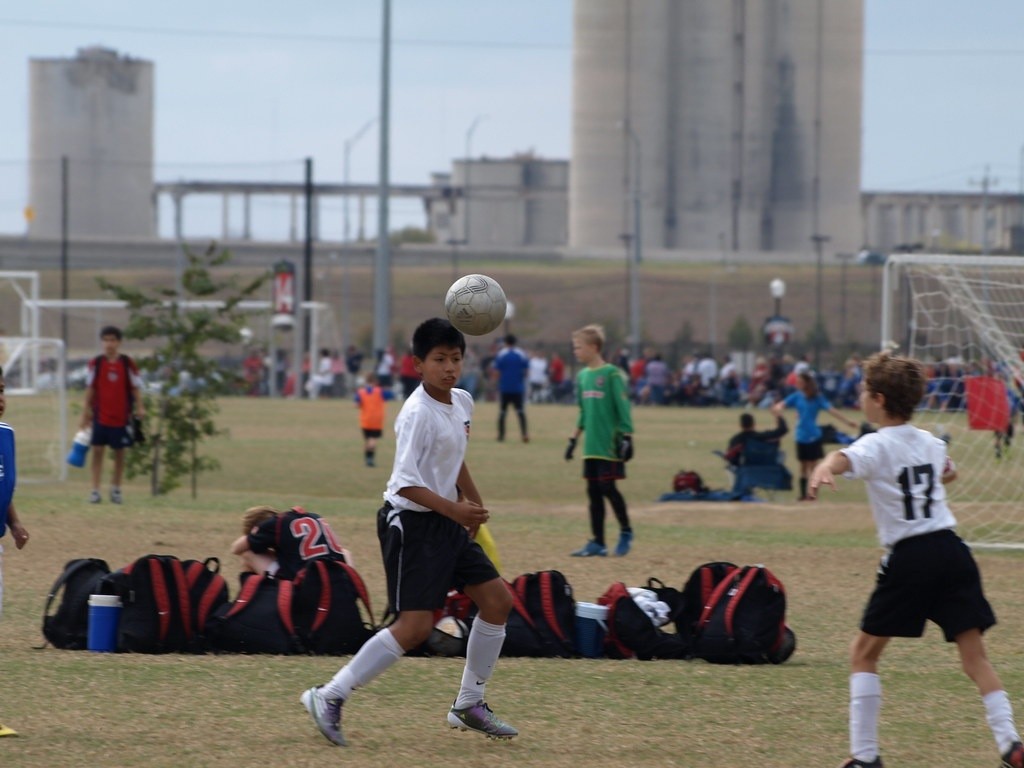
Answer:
[963,376,1009,431]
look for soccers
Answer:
[443,272,509,337]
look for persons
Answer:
[772,370,858,500]
[492,333,531,442]
[724,408,789,467]
[992,363,1015,457]
[230,505,354,595]
[454,485,507,584]
[807,355,1024,768]
[563,325,635,558]
[0,367,30,615]
[298,318,517,750]
[80,325,143,503]
[31,338,1024,453]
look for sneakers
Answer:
[571,539,608,556]
[447,696,519,741]
[615,529,634,555]
[834,756,884,768]
[1000,741,1024,768]
[297,683,346,749]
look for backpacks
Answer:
[405,567,579,659]
[700,557,795,667]
[108,553,195,654]
[602,572,690,661]
[182,555,229,653]
[294,557,376,656]
[38,558,112,651]
[221,568,298,656]
[681,561,740,665]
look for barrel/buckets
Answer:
[65,426,93,467]
[86,594,123,651]
[573,601,610,658]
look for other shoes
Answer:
[111,489,122,503]
[88,489,101,503]
